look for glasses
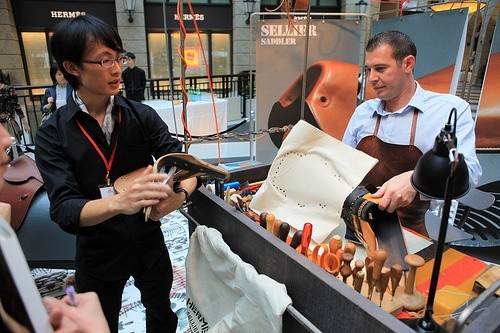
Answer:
[81,55,130,70]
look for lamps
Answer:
[398,107,450,333]
[243,0,256,25]
[122,0,135,23]
[355,0,368,21]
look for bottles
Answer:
[187,87,202,102]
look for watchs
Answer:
[175,187,187,201]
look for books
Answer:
[114,153,230,223]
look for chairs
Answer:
[424,189,495,242]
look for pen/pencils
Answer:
[66,286,79,308]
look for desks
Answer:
[141,99,228,137]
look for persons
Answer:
[40,63,75,114]
[122,52,146,103]
[340,30,483,240]
[33,15,198,333]
[0,69,31,161]
[0,120,112,333]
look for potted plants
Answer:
[240,78,256,119]
